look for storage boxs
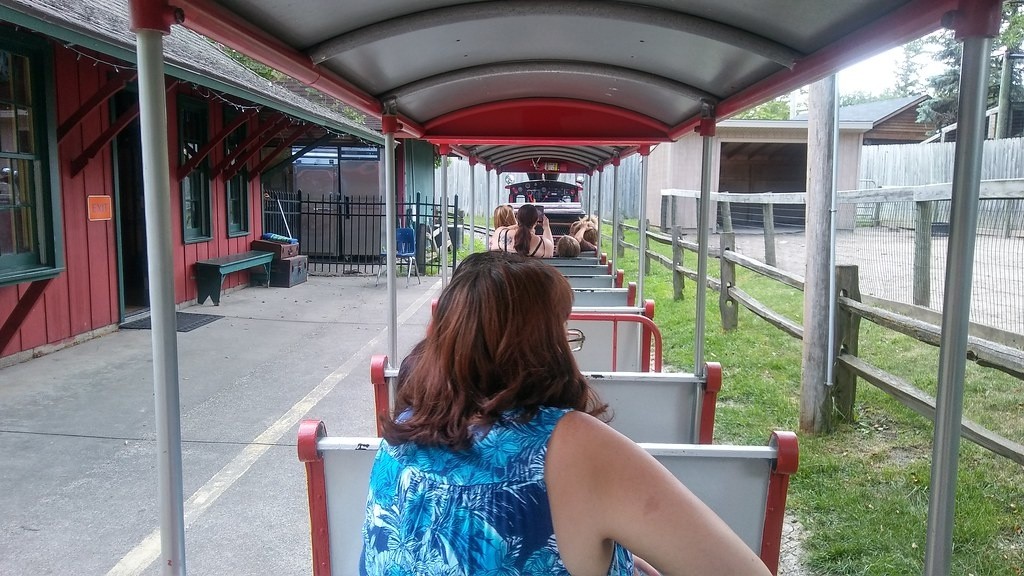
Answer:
[252,238,299,258]
[270,254,308,287]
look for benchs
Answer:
[297,242,799,576]
[195,252,275,305]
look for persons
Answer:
[360,248,774,576]
[489,204,599,258]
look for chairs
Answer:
[375,227,421,288]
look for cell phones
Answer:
[535,206,544,224]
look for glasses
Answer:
[537,220,543,224]
[566,329,585,352]
[496,205,514,210]
